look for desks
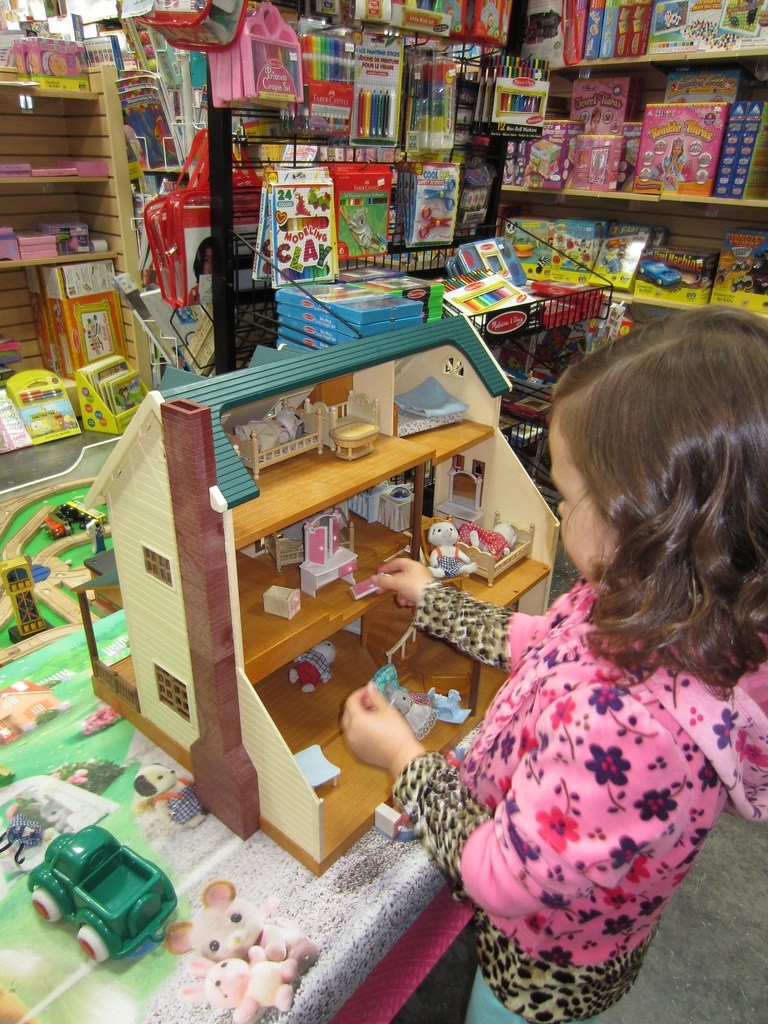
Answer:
[329,420,382,461]
[379,491,419,533]
[1,606,474,1024]
[347,480,413,525]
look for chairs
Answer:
[293,744,343,794]
[420,513,467,592]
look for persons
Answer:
[340,304,768,1024]
[188,237,212,305]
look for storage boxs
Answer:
[335,266,406,284]
[347,274,446,298]
[420,296,444,309]
[1,164,32,177]
[21,251,58,260]
[278,314,357,346]
[13,37,31,81]
[501,1,768,322]
[423,306,443,318]
[278,303,425,338]
[16,231,57,245]
[23,265,63,374]
[275,283,428,325]
[32,168,78,176]
[40,260,133,382]
[277,326,326,350]
[275,336,313,353]
[37,222,88,254]
[57,160,109,177]
[19,243,57,252]
[26,37,91,92]
[423,316,442,323]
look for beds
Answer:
[394,391,466,437]
[262,585,302,621]
[263,521,355,573]
[303,390,380,450]
[225,396,324,480]
[453,508,539,585]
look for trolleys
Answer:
[393,209,613,520]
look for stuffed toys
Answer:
[236,407,305,453]
[289,640,336,693]
[427,522,477,579]
[458,522,516,561]
[382,683,438,740]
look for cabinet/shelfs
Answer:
[214,404,562,875]
[0,59,155,464]
[434,466,485,530]
[498,0,768,338]
[299,510,358,598]
